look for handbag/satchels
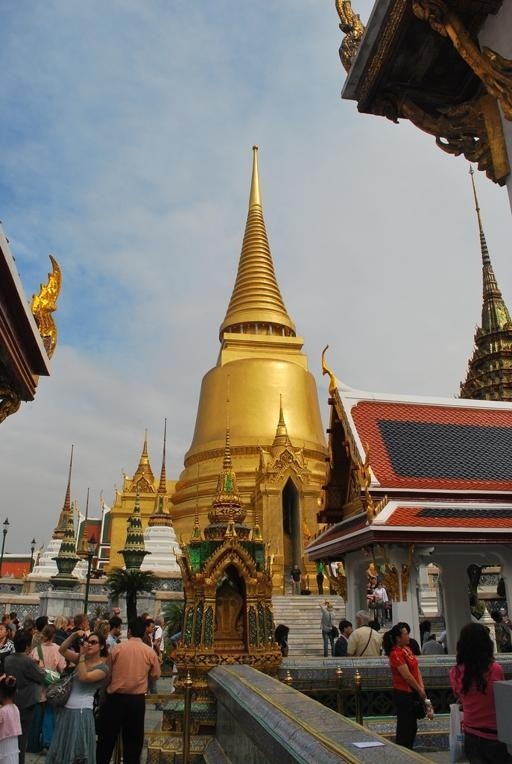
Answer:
[43,669,72,706]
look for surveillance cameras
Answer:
[416,547,434,556]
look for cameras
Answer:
[79,634,88,644]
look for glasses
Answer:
[89,640,98,645]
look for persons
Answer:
[1,608,183,761]
[274,622,290,657]
[314,560,511,762]
[292,563,303,596]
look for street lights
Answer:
[0,516,12,576]
[82,533,100,614]
[29,535,36,573]
